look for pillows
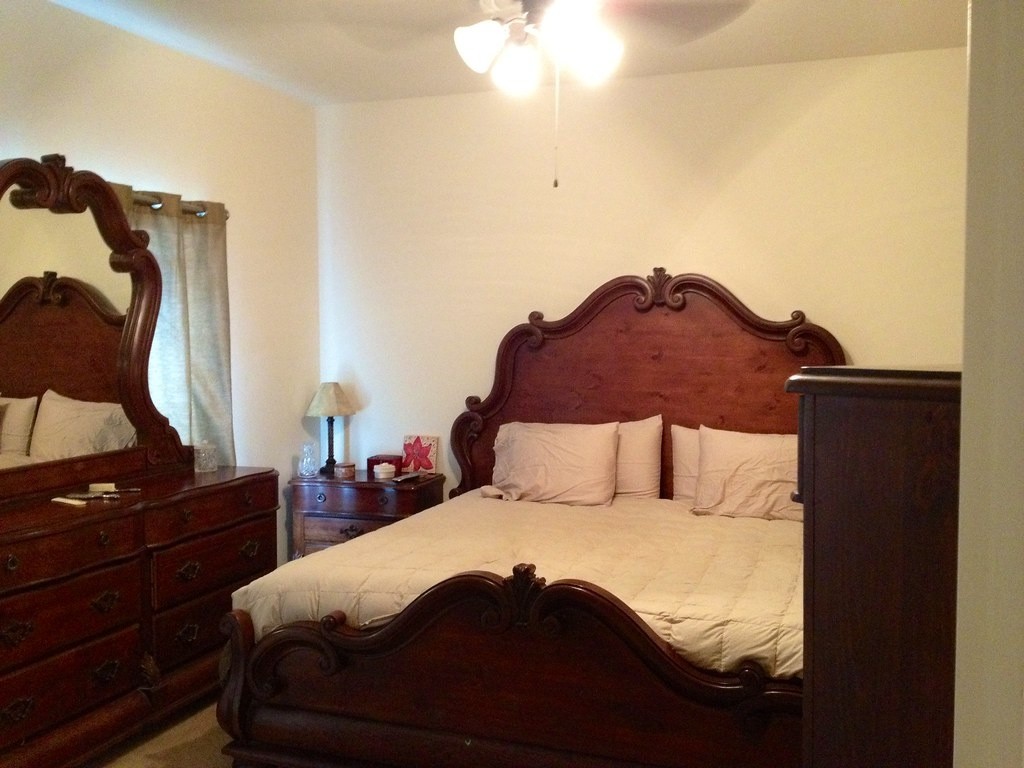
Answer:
[614,414,663,499]
[671,424,700,502]
[0,396,39,456]
[688,424,804,522]
[480,422,620,506]
[30,389,137,462]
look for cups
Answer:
[193,441,218,472]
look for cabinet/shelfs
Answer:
[782,364,962,768]
[0,464,280,768]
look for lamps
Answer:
[307,382,356,473]
[453,0,624,188]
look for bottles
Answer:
[296,442,318,478]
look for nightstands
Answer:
[287,469,446,560]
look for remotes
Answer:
[392,473,421,483]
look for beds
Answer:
[0,272,142,471]
[215,266,849,768]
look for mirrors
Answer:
[0,152,195,501]
